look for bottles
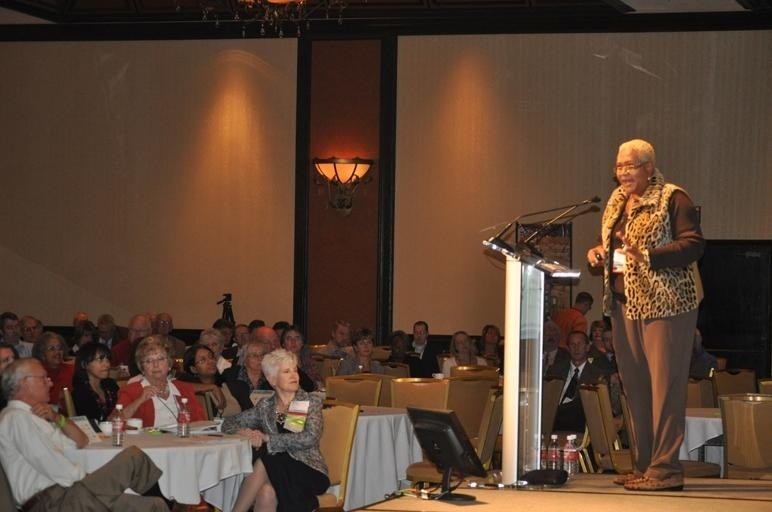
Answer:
[563,435,577,481]
[548,434,561,470]
[111,403,125,447]
[177,398,191,438]
[572,434,579,474]
[540,433,548,470]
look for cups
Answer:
[99,421,113,435]
[431,373,445,379]
[126,417,143,434]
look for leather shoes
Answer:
[613,473,637,485]
[168,496,211,512]
[624,476,684,491]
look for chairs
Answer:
[325,378,383,407]
[259,402,360,512]
[447,363,501,383]
[713,368,761,396]
[193,390,216,423]
[578,380,633,474]
[687,376,718,409]
[575,420,595,473]
[406,390,503,489]
[389,379,450,411]
[541,379,565,447]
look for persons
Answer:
[587,139,704,490]
[549,332,606,431]
[184,345,253,419]
[21,316,43,342]
[73,342,119,428]
[543,321,571,380]
[336,328,385,375]
[118,336,206,427]
[0,359,191,512]
[689,330,717,381]
[233,325,250,345]
[589,320,604,351]
[72,311,87,329]
[237,345,272,390]
[153,312,183,353]
[248,319,266,333]
[273,321,289,340]
[407,321,435,360]
[478,325,504,372]
[322,319,353,356]
[34,331,74,402]
[68,321,96,354]
[0,312,33,358]
[109,314,156,366]
[252,327,281,352]
[91,314,123,350]
[282,327,326,392]
[555,292,593,350]
[195,328,231,373]
[222,349,330,512]
[383,331,432,377]
[442,331,486,378]
[602,332,616,375]
[0,345,17,373]
[212,318,232,348]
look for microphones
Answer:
[489,195,601,241]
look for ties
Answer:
[559,367,580,405]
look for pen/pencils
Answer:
[208,434,224,438]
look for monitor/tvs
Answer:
[406,405,487,502]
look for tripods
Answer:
[215,292,235,324]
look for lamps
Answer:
[310,154,373,217]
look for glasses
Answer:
[23,374,47,383]
[159,320,169,325]
[140,355,168,365]
[47,343,64,352]
[613,160,649,174]
[193,358,206,366]
